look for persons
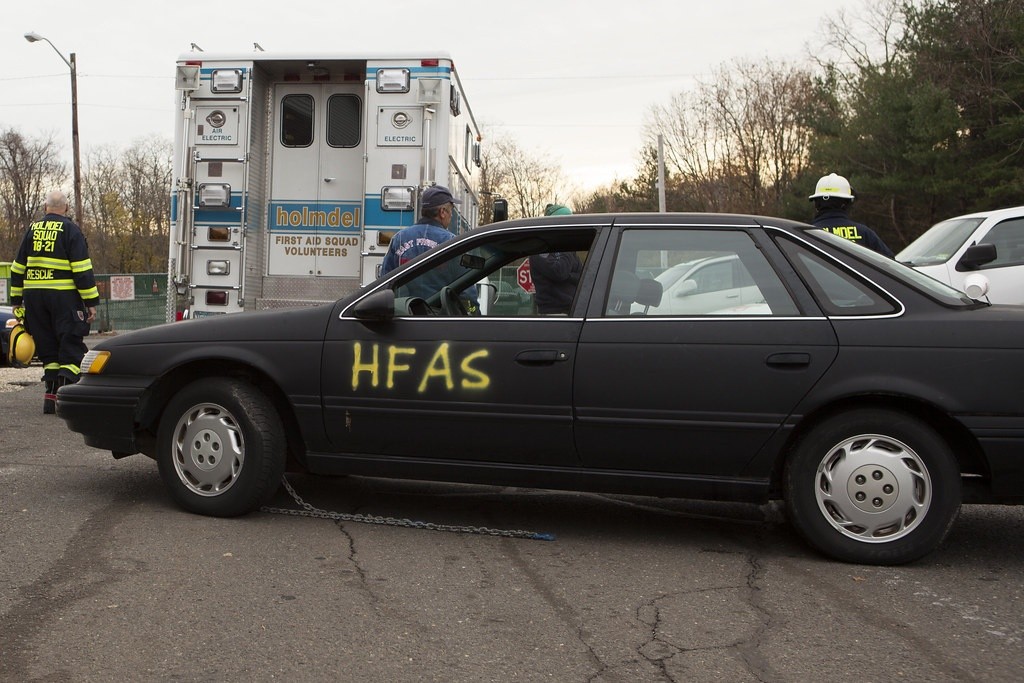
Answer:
[807,173,895,261]
[526,204,583,315]
[378,185,483,318]
[10,191,100,416]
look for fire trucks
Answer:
[162,37,484,328]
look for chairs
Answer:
[606,270,664,316]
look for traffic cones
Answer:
[152,278,159,293]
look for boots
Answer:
[43,376,72,414]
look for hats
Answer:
[421,185,463,207]
[544,204,572,216]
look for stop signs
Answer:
[517,258,537,294]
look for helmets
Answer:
[809,173,855,198]
[9,323,36,368]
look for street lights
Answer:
[24,32,83,233]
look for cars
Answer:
[630,252,853,319]
[55,211,1024,566]
[837,205,1024,308]
